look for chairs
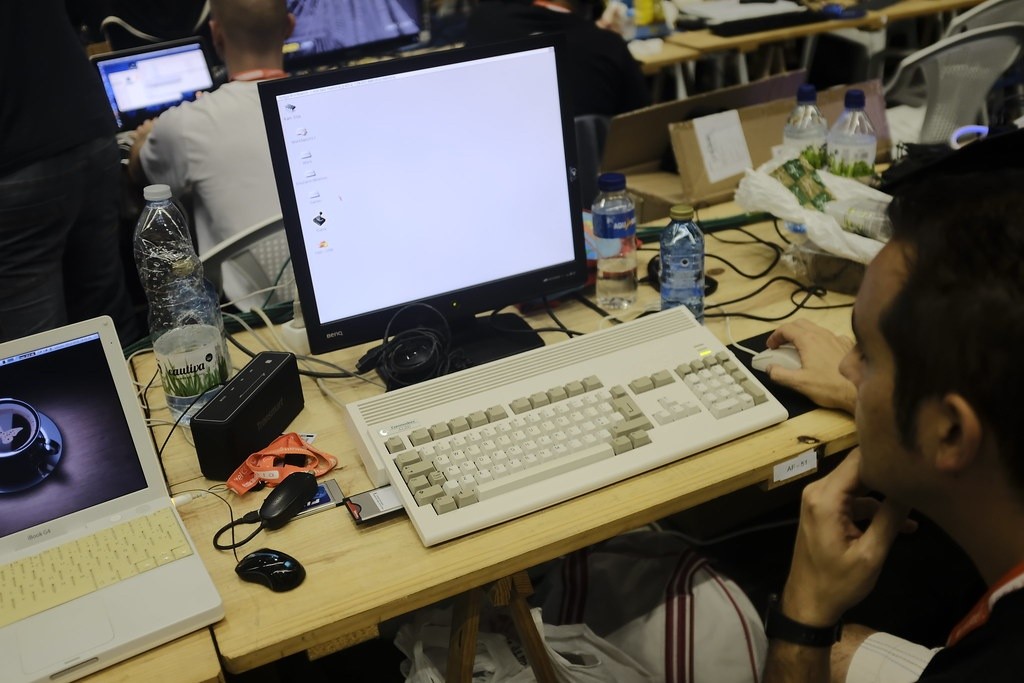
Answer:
[882,20,1024,144]
[941,0,1024,40]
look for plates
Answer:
[1,411,63,495]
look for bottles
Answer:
[132,185,234,428]
[622,2,637,41]
[826,89,877,188]
[592,173,638,312]
[782,87,829,233]
[659,205,706,328]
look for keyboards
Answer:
[344,304,788,546]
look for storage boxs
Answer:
[598,67,894,225]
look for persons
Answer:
[134,0,334,314]
[764,128,1023,683]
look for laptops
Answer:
[89,34,229,148]
[0,315,226,683]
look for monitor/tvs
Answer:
[257,26,589,390]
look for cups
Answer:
[1,397,40,459]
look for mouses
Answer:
[752,342,803,372]
[235,548,306,593]
[389,340,437,373]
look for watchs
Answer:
[763,592,844,647]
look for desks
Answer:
[625,37,703,102]
[121,219,860,683]
[666,0,985,85]
[66,356,227,683]
